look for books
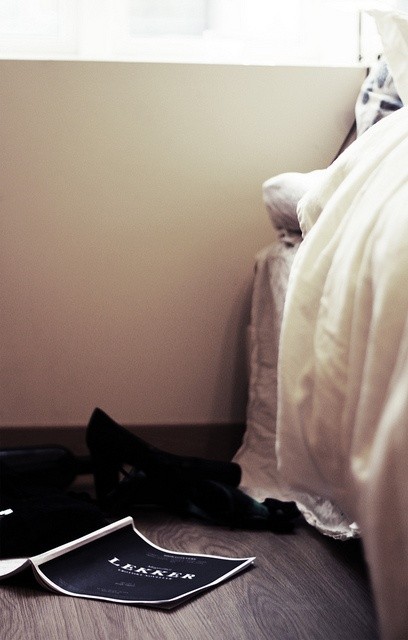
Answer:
[1,515,255,611]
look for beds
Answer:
[233,10,408,640]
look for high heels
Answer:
[86,408,241,517]
